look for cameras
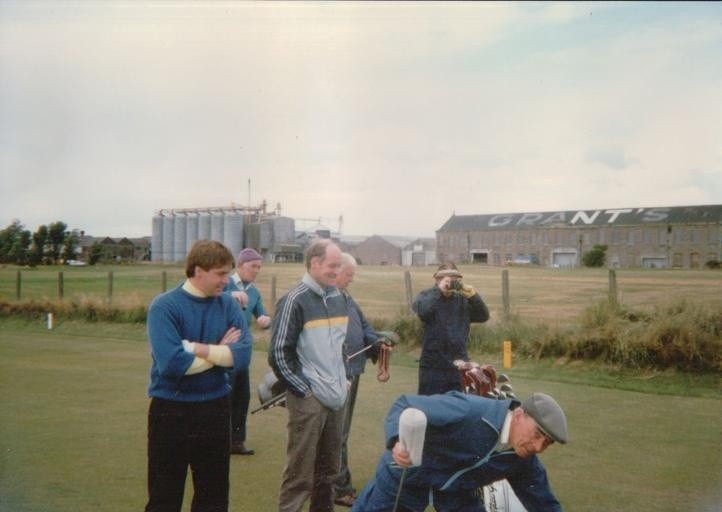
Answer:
[446,280,461,290]
[240,303,248,312]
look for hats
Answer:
[433,263,462,278]
[237,248,260,264]
[520,392,566,444]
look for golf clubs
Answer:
[250,331,399,414]
[453,360,516,400]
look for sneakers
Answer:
[335,493,356,506]
[230,444,255,455]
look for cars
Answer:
[66,259,86,267]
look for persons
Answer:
[144,239,253,511]
[335,252,400,504]
[268,238,351,511]
[349,389,568,512]
[411,261,490,394]
[222,247,274,453]
